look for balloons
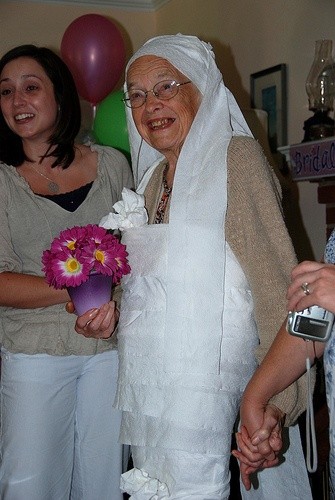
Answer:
[60,13,125,108]
[90,91,132,160]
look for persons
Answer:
[240,226,335,500]
[0,44,137,500]
[63,33,319,500]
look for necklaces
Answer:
[31,165,61,194]
[153,162,173,225]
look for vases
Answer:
[67,271,112,317]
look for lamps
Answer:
[303,40,335,143]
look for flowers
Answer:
[41,224,131,292]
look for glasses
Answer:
[121,79,192,109]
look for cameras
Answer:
[286,305,335,341]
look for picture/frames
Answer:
[250,64,287,154]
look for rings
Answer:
[301,282,309,295]
